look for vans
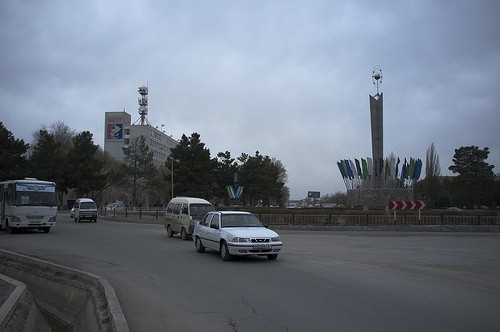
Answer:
[164,196,215,240]
[74,198,97,223]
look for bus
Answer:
[0,178,58,234]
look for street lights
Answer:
[169,146,174,199]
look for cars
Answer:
[104,202,124,213]
[192,211,283,261]
[446,207,462,212]
[70,206,74,218]
